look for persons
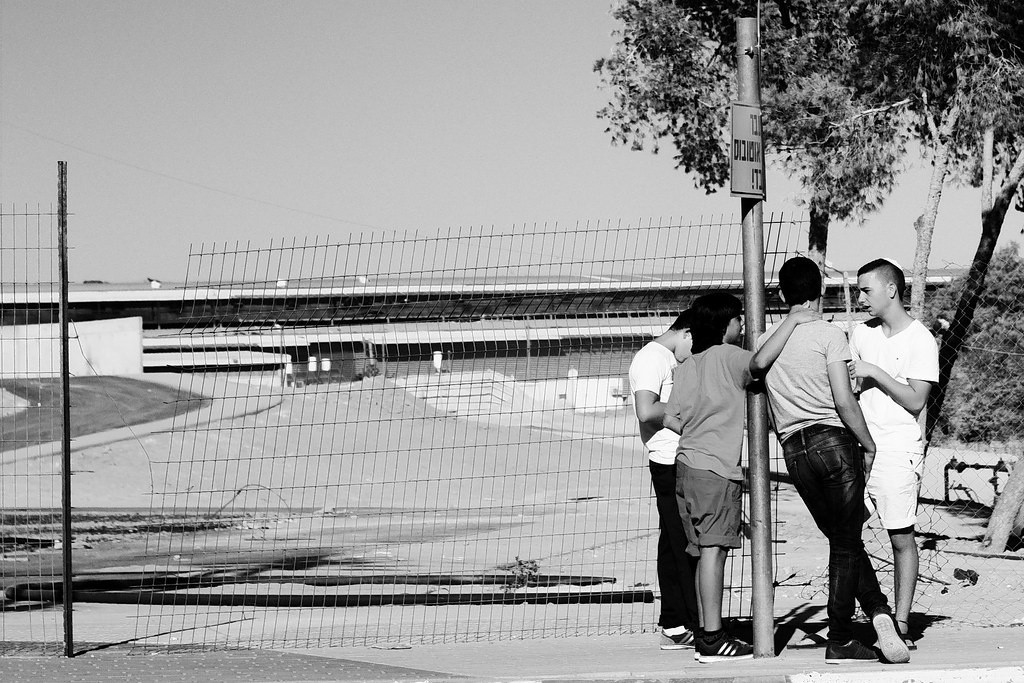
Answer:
[664,291,821,662]
[757,257,911,664]
[627,309,724,650]
[847,258,939,650]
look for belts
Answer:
[781,424,836,448]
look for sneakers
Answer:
[694,632,753,662]
[825,639,879,663]
[660,629,695,649]
[873,607,910,663]
